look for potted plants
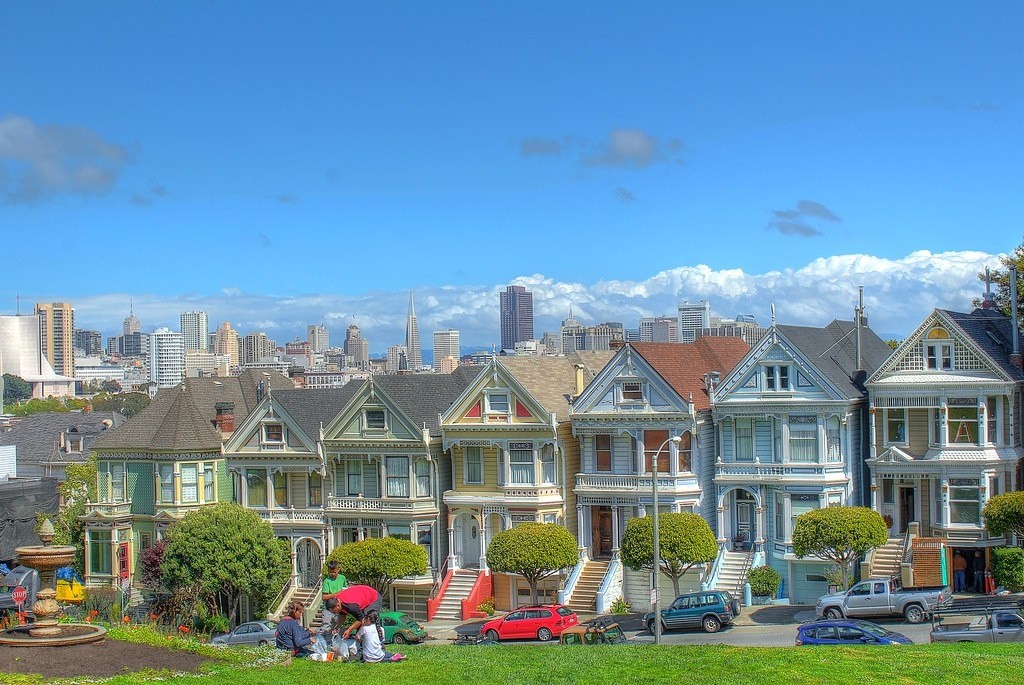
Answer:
[882,515,893,538]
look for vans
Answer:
[557,616,626,645]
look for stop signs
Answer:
[11,586,27,603]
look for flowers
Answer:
[734,536,744,551]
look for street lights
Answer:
[652,436,683,645]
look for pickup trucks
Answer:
[930,610,1024,644]
[815,574,954,624]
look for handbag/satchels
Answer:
[348,641,358,656]
[312,631,328,654]
[332,634,349,662]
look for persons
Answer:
[953,550,967,593]
[275,601,316,658]
[322,561,348,632]
[326,585,382,640]
[355,609,409,663]
[973,551,984,594]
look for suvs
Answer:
[794,618,914,646]
[643,590,741,637]
[478,603,579,642]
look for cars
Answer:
[348,612,428,645]
[212,620,280,646]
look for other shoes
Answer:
[390,653,402,662]
